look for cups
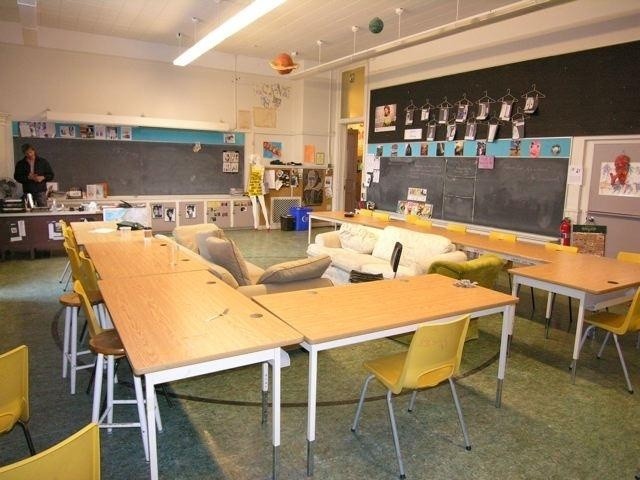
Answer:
[168,245,180,265]
[143,227,153,240]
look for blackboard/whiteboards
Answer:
[366,156,569,239]
[13,137,245,194]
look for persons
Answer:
[382,105,396,127]
[301,170,323,207]
[187,205,195,218]
[13,144,53,207]
[167,209,173,221]
[154,207,159,217]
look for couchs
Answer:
[307,229,466,285]
[153,232,333,300]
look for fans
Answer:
[0,177,18,200]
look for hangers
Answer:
[403,83,547,126]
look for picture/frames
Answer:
[261,140,284,162]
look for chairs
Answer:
[415,219,433,227]
[447,222,467,232]
[1,345,40,458]
[372,211,391,221]
[405,214,420,222]
[543,241,579,253]
[354,210,372,217]
[569,284,640,397]
[616,250,640,264]
[353,314,473,478]
[57,221,163,464]
[488,230,518,242]
[2,422,99,479]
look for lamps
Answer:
[287,0,550,81]
[173,0,287,67]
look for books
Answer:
[86,182,108,198]
[397,187,433,219]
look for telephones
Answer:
[117,221,143,230]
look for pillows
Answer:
[172,223,332,287]
[339,223,453,267]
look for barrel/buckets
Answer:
[280,214,295,230]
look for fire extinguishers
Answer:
[560,217,570,246]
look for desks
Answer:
[251,273,520,480]
[84,239,209,280]
[97,270,303,480]
[506,254,639,385]
[305,210,582,339]
[0,210,103,261]
